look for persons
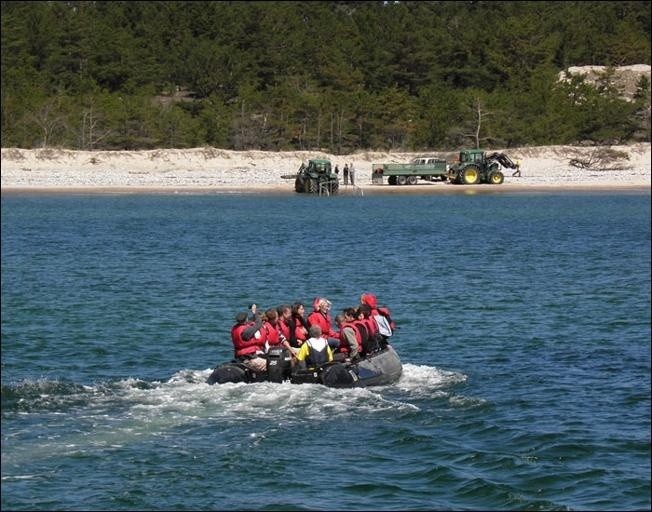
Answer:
[512,160,521,177]
[335,164,339,174]
[343,164,349,185]
[349,162,354,185]
[230,293,393,375]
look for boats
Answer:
[214,344,403,388]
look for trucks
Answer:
[372,149,503,185]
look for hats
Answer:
[361,293,376,308]
[236,312,248,322]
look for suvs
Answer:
[295,160,338,192]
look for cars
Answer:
[413,157,443,165]
